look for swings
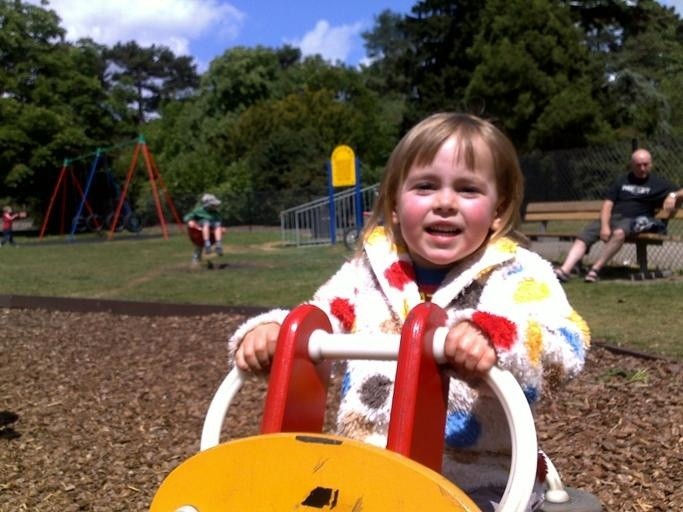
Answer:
[71,144,142,233]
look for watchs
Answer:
[667,192,678,201]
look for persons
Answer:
[0,205,27,248]
[183,193,226,256]
[227,111,591,510]
[553,148,683,282]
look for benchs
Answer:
[524,201,663,268]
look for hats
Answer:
[202,194,221,206]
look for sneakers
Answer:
[585,269,599,282]
[552,266,569,281]
